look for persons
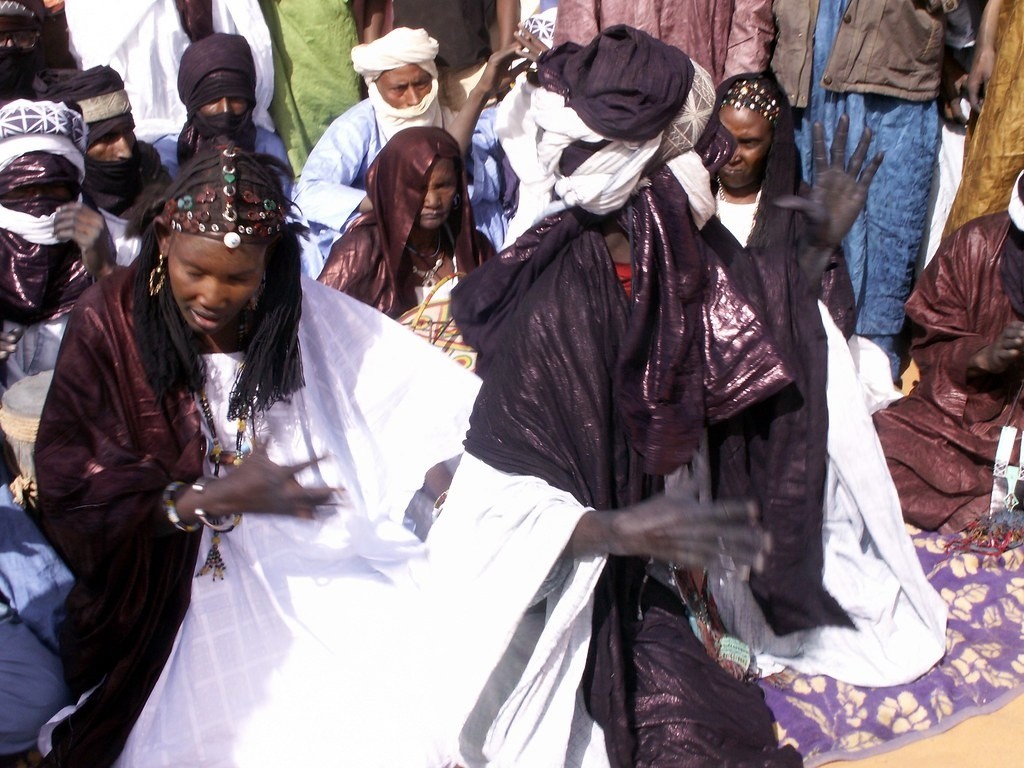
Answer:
[1,0,522,389]
[296,26,463,263]
[315,126,499,381]
[553,0,772,82]
[449,28,552,246]
[873,170,1024,536]
[942,0,1024,242]
[413,27,886,768]
[32,140,485,768]
[771,0,970,384]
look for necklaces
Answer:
[416,232,441,258]
[405,251,445,286]
[197,382,247,582]
[717,177,728,202]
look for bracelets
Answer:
[164,481,200,532]
[192,475,232,531]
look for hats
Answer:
[178,33,256,103]
[31,64,136,148]
[350,26,439,85]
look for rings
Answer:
[538,51,542,56]
